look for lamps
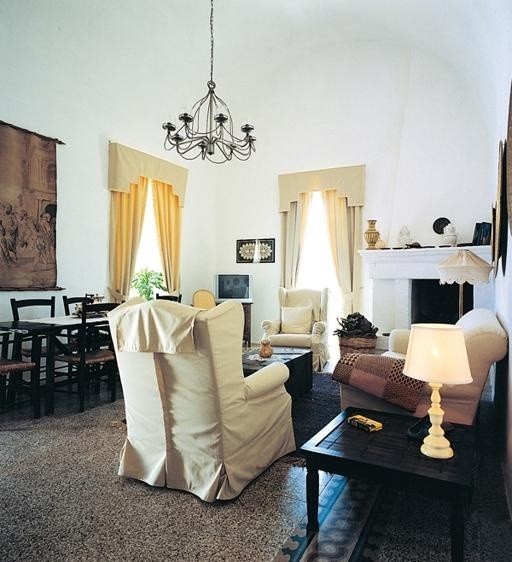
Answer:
[437,246,495,321]
[161,105,257,165]
[403,321,474,463]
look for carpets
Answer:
[118,371,340,467]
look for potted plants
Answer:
[332,310,379,358]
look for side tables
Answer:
[301,406,478,562]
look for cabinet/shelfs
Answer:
[216,302,253,346]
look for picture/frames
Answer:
[488,81,511,277]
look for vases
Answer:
[364,218,379,248]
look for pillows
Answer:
[280,304,313,334]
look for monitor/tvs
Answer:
[214,272,254,305]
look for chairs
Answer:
[192,289,215,310]
[327,306,507,428]
[0,294,184,418]
[260,287,328,375]
[108,295,295,504]
